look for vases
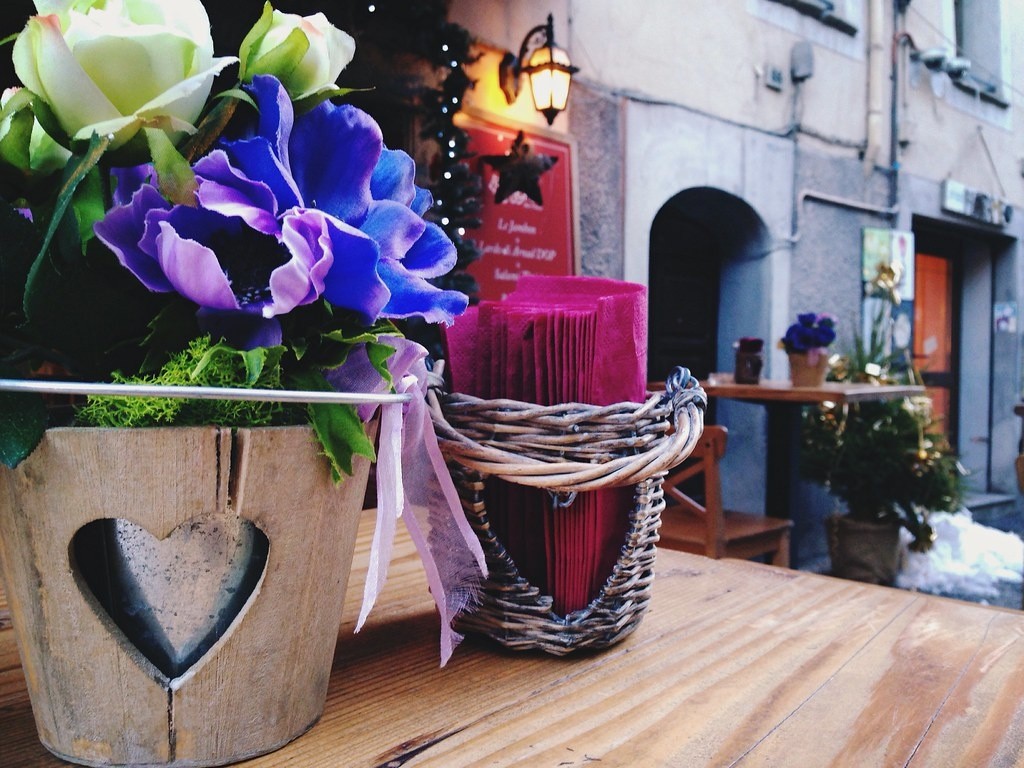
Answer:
[0,420,382,768]
[790,352,824,388]
[735,352,762,384]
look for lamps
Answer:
[497,10,579,127]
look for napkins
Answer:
[440,273,648,607]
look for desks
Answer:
[0,507,1024,768]
[649,377,928,405]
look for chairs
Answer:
[657,425,795,572]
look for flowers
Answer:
[0,0,466,462]
[734,336,765,353]
[780,312,837,351]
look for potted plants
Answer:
[800,289,970,586]
